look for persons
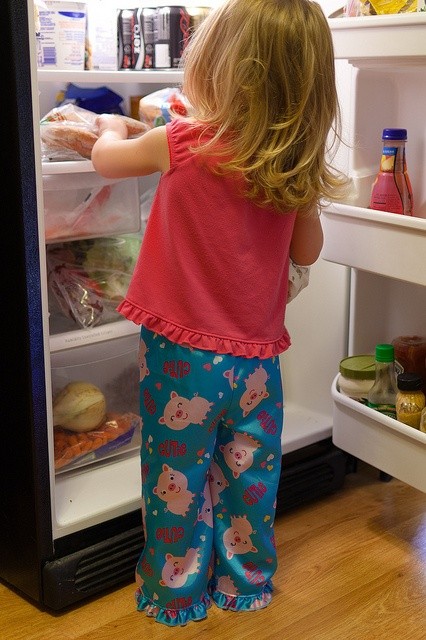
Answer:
[90,0,335,625]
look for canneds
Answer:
[189,7,214,37]
[131,8,155,71]
[117,9,133,70]
[153,8,189,69]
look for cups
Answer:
[393,334,425,370]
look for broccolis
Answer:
[83,233,145,307]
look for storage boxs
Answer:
[39,171,142,243]
[48,330,143,479]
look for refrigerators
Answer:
[1,0,426,619]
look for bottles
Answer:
[339,356,391,402]
[369,128,414,217]
[369,343,399,420]
[394,371,423,426]
